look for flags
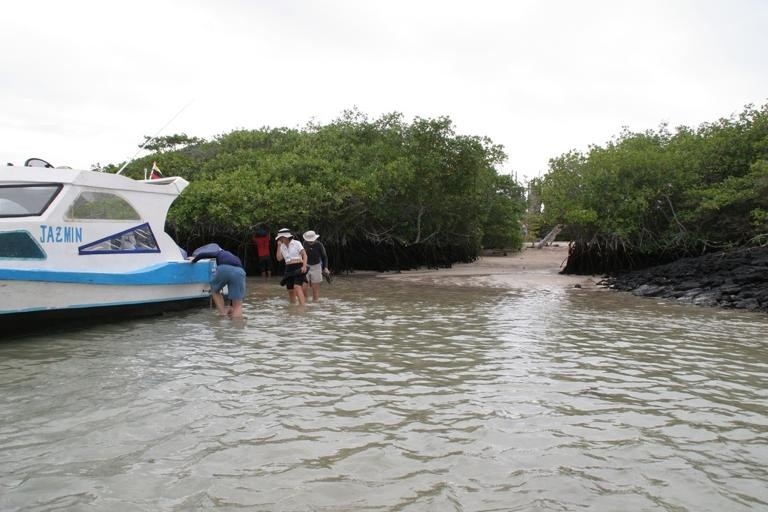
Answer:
[152,166,163,179]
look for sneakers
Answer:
[321,271,333,285]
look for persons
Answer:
[522,223,528,236]
[252,230,272,280]
[304,230,329,302]
[191,248,246,318]
[275,228,308,305]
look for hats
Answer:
[303,231,320,241]
[258,230,266,235]
[275,228,293,241]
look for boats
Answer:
[0,158,229,320]
